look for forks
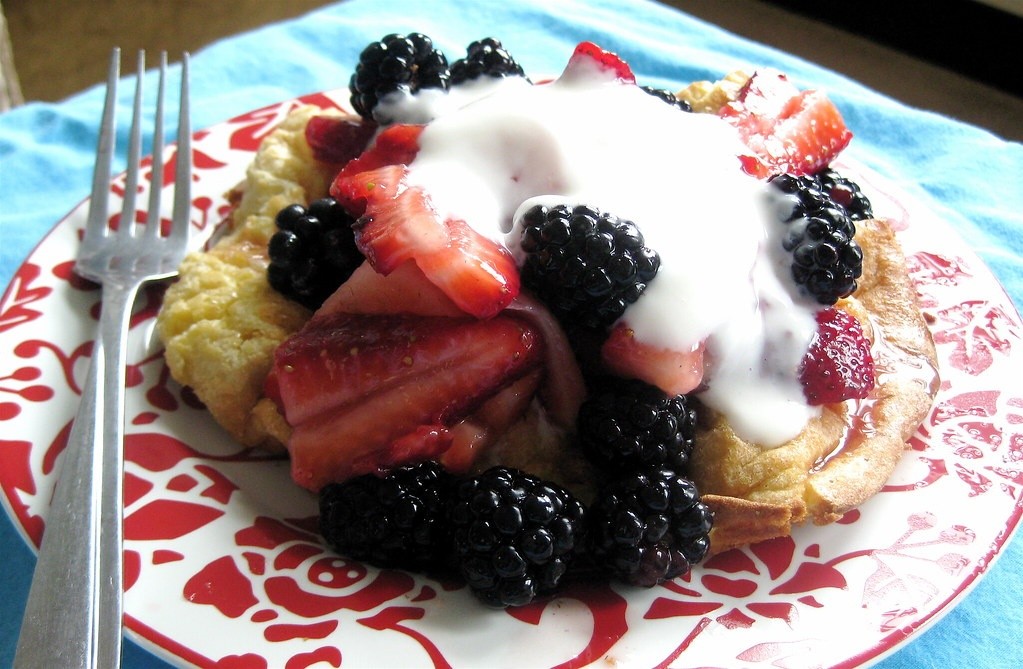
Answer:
[11,50,191,669]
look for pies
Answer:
[154,100,939,558]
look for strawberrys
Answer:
[254,39,877,492]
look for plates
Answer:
[0,74,1022,669]
